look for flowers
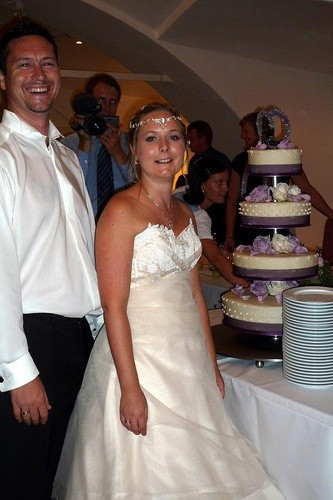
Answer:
[245,182,311,202]
[232,280,299,303]
[251,233,307,255]
[317,255,333,288]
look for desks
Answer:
[206,309,333,500]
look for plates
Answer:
[282,286,333,389]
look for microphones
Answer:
[72,92,98,116]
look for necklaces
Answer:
[139,183,177,230]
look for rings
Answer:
[125,420,132,424]
[21,411,30,416]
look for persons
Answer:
[0,26,104,500]
[57,73,333,288]
[51,102,285,500]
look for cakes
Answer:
[238,182,311,227]
[221,280,300,332]
[247,108,303,174]
[232,232,320,278]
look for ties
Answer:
[97,141,113,219]
